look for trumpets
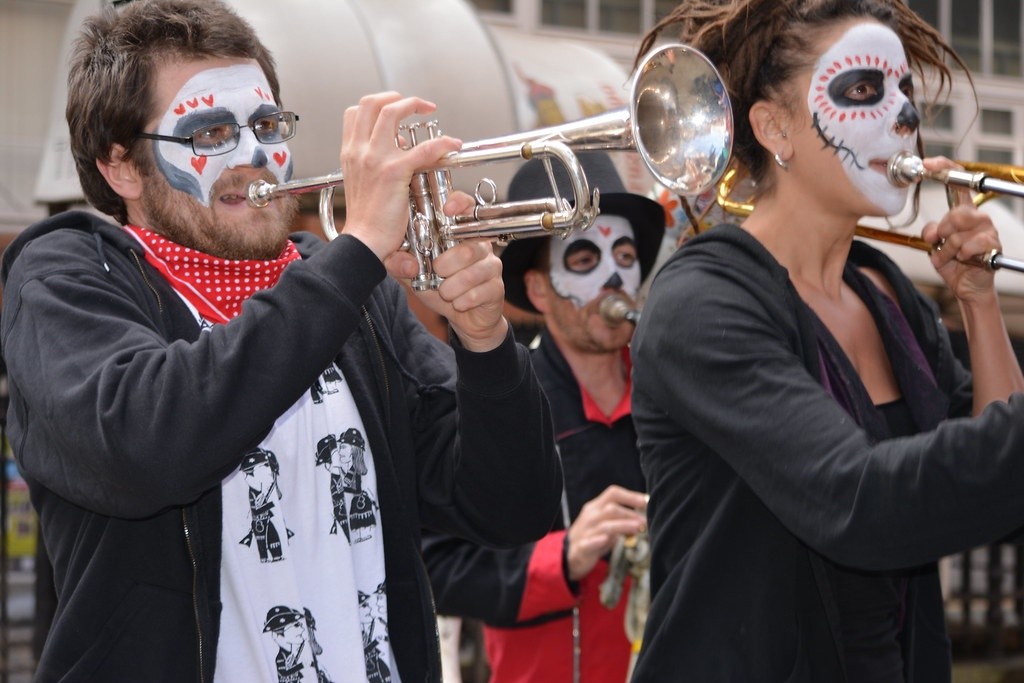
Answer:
[714,147,1024,273]
[246,44,736,296]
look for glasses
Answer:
[137,111,298,157]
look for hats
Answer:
[496,151,664,314]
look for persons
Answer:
[631,0,1024,683]
[0,0,665,683]
